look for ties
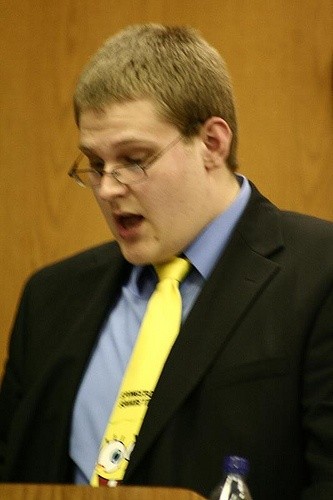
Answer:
[88,255,192,488]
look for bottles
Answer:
[208,454,253,500]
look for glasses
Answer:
[68,131,187,190]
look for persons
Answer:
[1,24,333,499]
